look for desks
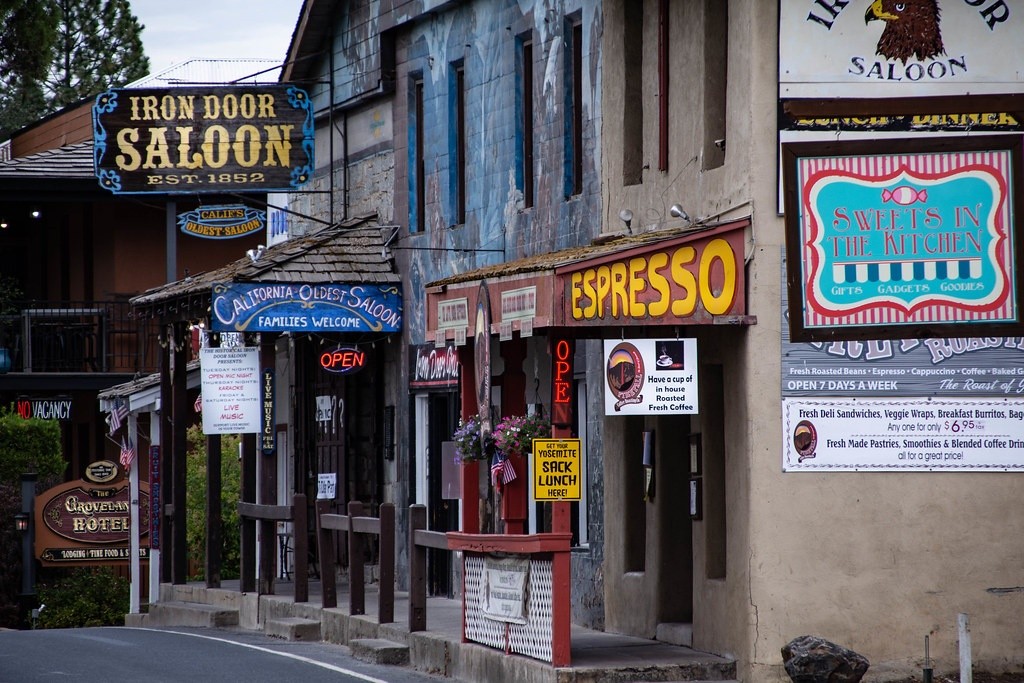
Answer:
[33,322,99,374]
[276,533,319,581]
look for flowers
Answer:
[492,415,550,456]
[453,416,480,465]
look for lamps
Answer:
[618,209,633,234]
[15,510,29,537]
[671,204,688,220]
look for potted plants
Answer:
[0,273,23,374]
